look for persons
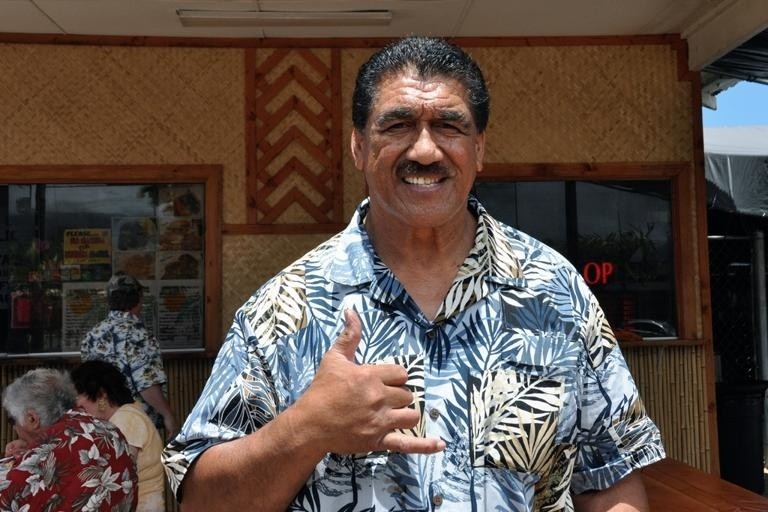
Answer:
[80,274,177,443]
[157,33,669,512]
[5,359,167,512]
[0,367,139,512]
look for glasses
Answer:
[8,415,24,427]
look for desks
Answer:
[641,456,768,512]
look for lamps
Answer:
[177,10,392,25]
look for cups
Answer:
[0,456,17,470]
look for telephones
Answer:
[626,318,675,337]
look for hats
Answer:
[106,272,150,297]
